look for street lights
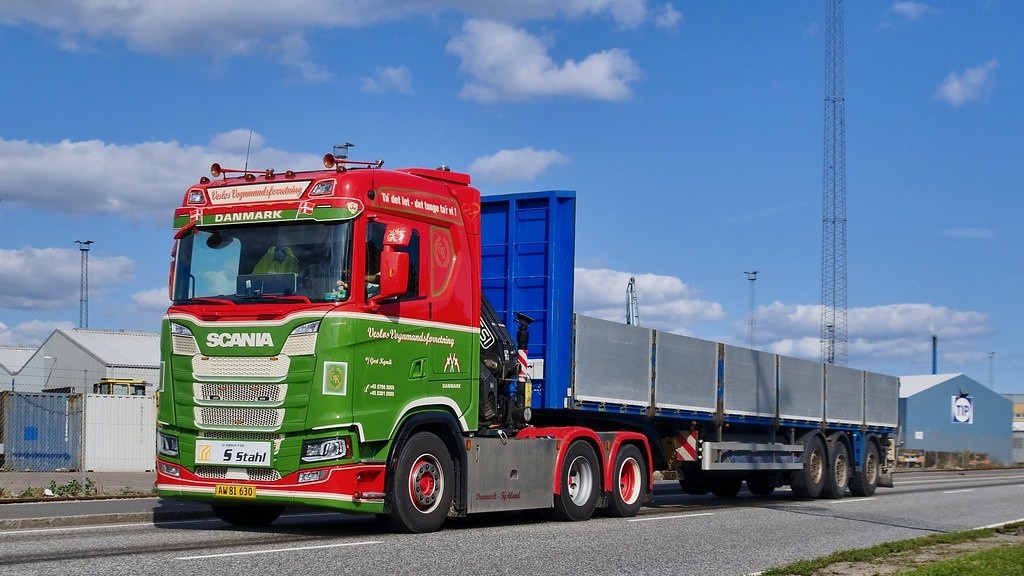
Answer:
[743,271,760,351]
[74,239,94,329]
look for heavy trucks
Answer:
[155,142,902,535]
